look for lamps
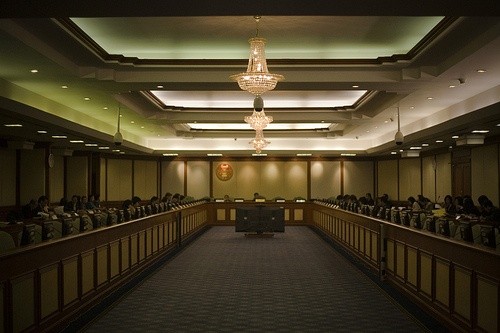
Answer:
[395,104,404,145]
[244,107,274,156]
[226,16,286,97]
[114,102,123,147]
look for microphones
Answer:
[114,132,123,145]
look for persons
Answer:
[336,192,500,227]
[19,192,186,221]
[223,195,230,202]
[253,193,262,200]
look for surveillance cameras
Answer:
[395,132,404,145]
